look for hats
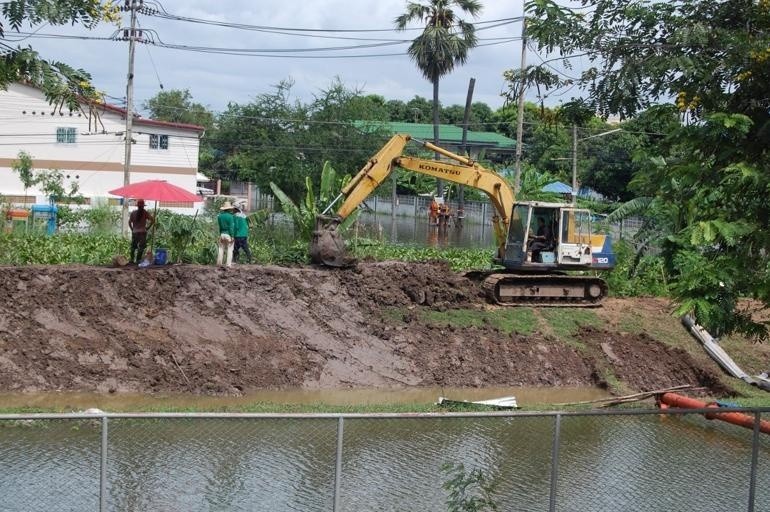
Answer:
[220,201,233,211]
[135,200,147,207]
[232,202,242,211]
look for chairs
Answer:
[541,221,557,252]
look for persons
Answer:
[128,200,154,264]
[216,201,235,266]
[529,216,552,262]
[233,207,253,264]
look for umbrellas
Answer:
[107,179,204,252]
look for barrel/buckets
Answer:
[154,249,166,265]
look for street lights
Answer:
[570,123,623,205]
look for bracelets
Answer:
[535,235,537,238]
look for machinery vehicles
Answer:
[307,133,617,309]
